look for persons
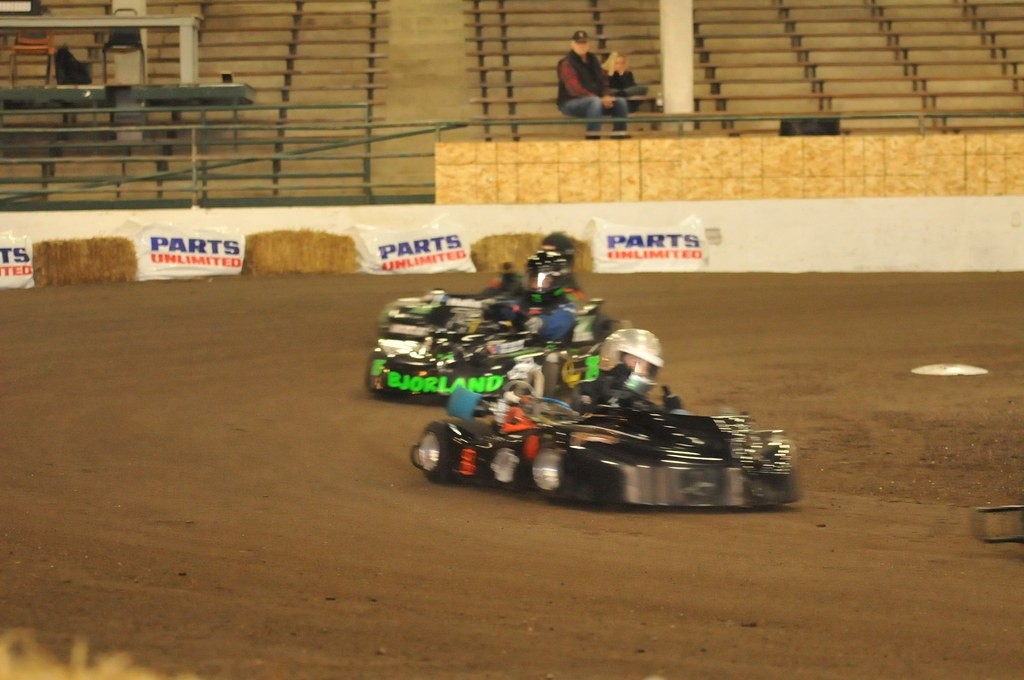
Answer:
[609,57,656,112]
[479,236,690,419]
[557,30,628,139]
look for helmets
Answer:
[523,250,575,302]
[600,328,663,397]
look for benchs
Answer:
[0,0,389,199]
[463,0,1024,132]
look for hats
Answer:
[572,31,590,42]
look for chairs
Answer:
[11,28,56,86]
[101,8,146,84]
